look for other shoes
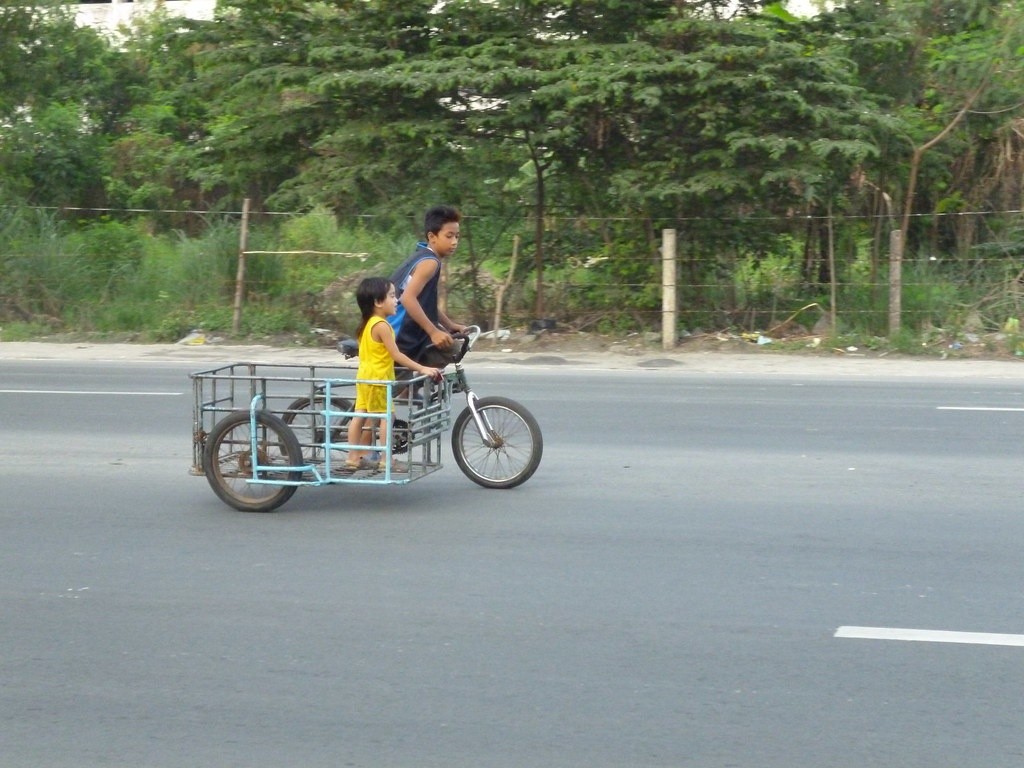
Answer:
[378,459,410,473]
[343,457,376,471]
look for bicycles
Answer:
[189,323,544,510]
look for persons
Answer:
[343,275,411,473]
[386,203,470,405]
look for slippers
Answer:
[360,451,381,462]
[393,393,426,406]
